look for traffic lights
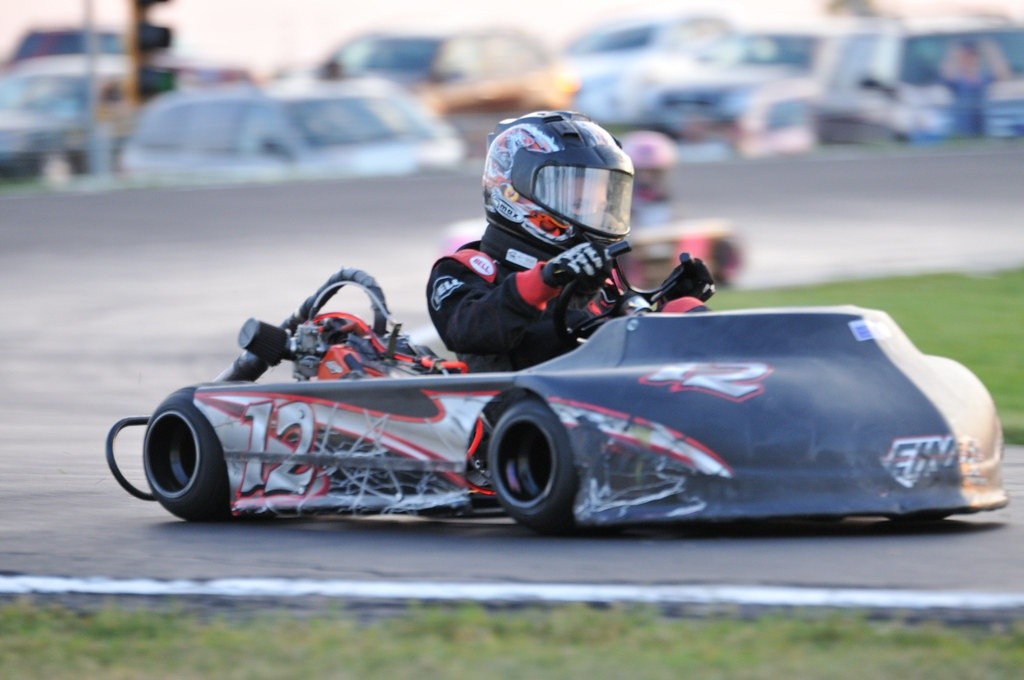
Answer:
[134,0,179,99]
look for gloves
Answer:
[658,253,715,303]
[542,242,613,292]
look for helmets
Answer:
[482,109,636,256]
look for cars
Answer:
[113,74,470,192]
[315,18,581,160]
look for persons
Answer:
[424,109,681,373]
[937,37,1011,137]
